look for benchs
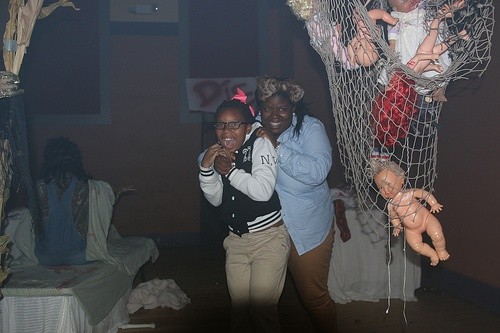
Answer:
[0,235,161,333]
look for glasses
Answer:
[214,121,247,130]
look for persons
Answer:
[197,100,290,333]
[369,160,450,267]
[326,172,357,242]
[7,135,136,267]
[290,0,471,145]
[250,79,342,333]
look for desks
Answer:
[330,206,421,304]
